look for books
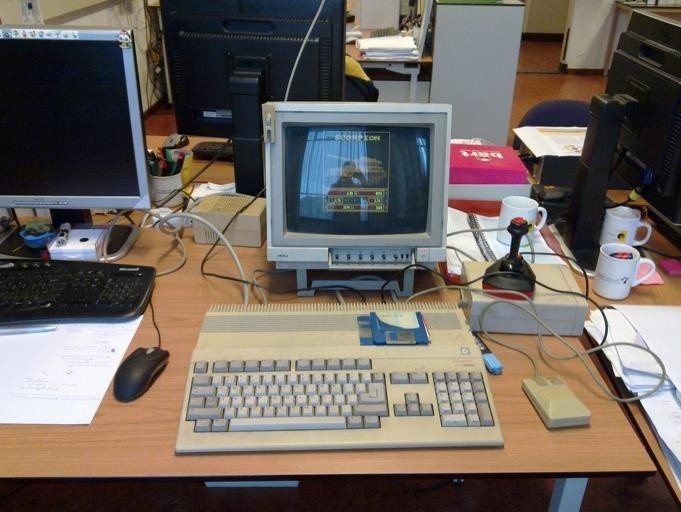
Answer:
[354,32,420,61]
[436,192,568,286]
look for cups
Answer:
[497,195,548,247]
[180,150,193,197]
[592,204,656,300]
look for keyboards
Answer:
[1,262,155,318]
[192,141,232,160]
[176,344,504,452]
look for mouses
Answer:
[523,374,592,429]
[112,347,170,403]
[162,134,189,149]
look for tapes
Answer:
[170,149,193,170]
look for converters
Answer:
[191,194,265,248]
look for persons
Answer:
[328,158,371,235]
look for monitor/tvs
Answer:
[0,24,151,261]
[158,0,346,198]
[263,101,452,296]
[548,8,681,278]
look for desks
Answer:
[1,135,681,512]
[344,28,432,103]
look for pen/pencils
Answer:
[146,147,184,176]
